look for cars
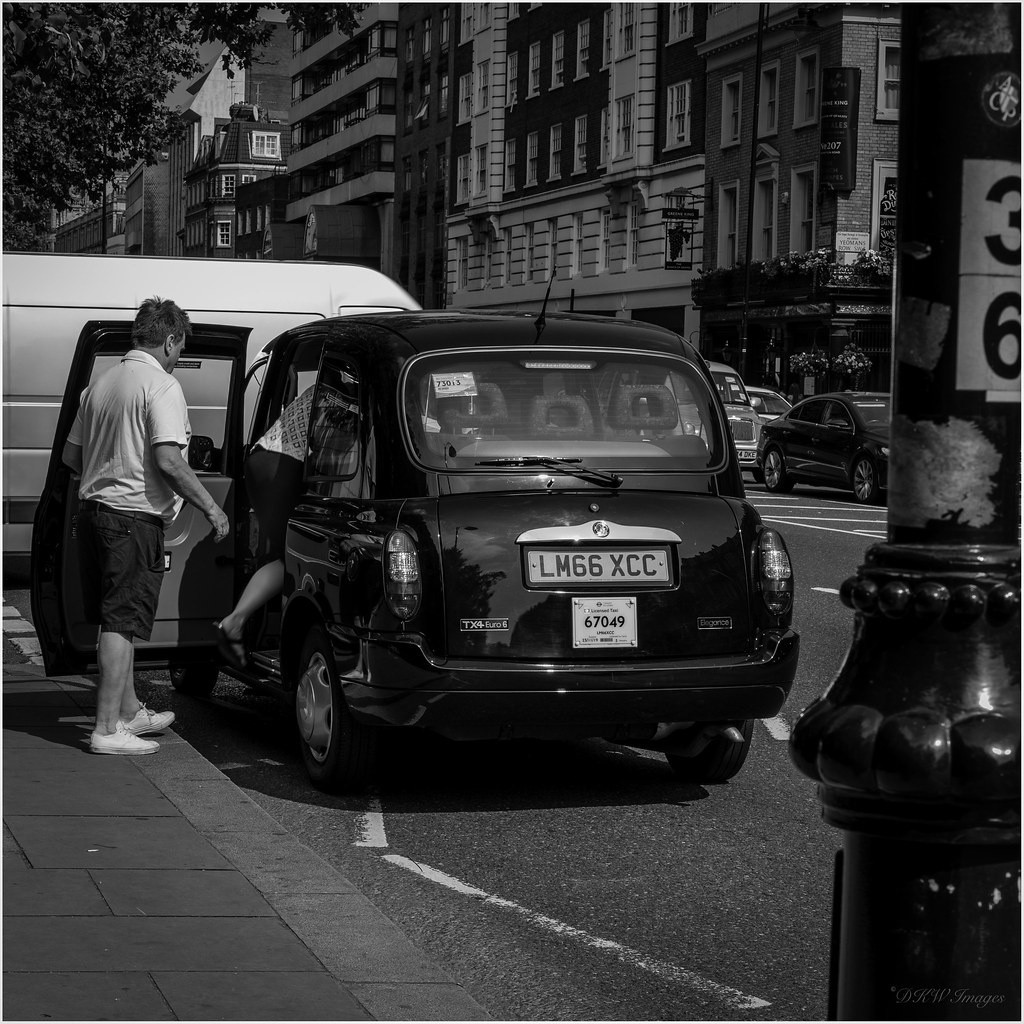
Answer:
[593,360,798,484]
[223,307,801,801]
[753,391,891,504]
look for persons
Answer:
[211,382,319,669]
[63,298,230,757]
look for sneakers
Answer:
[90,720,159,755]
[121,698,175,736]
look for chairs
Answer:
[432,373,682,440]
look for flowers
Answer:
[788,343,873,375]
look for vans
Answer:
[2,251,433,584]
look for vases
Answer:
[803,376,815,396]
[835,372,866,392]
